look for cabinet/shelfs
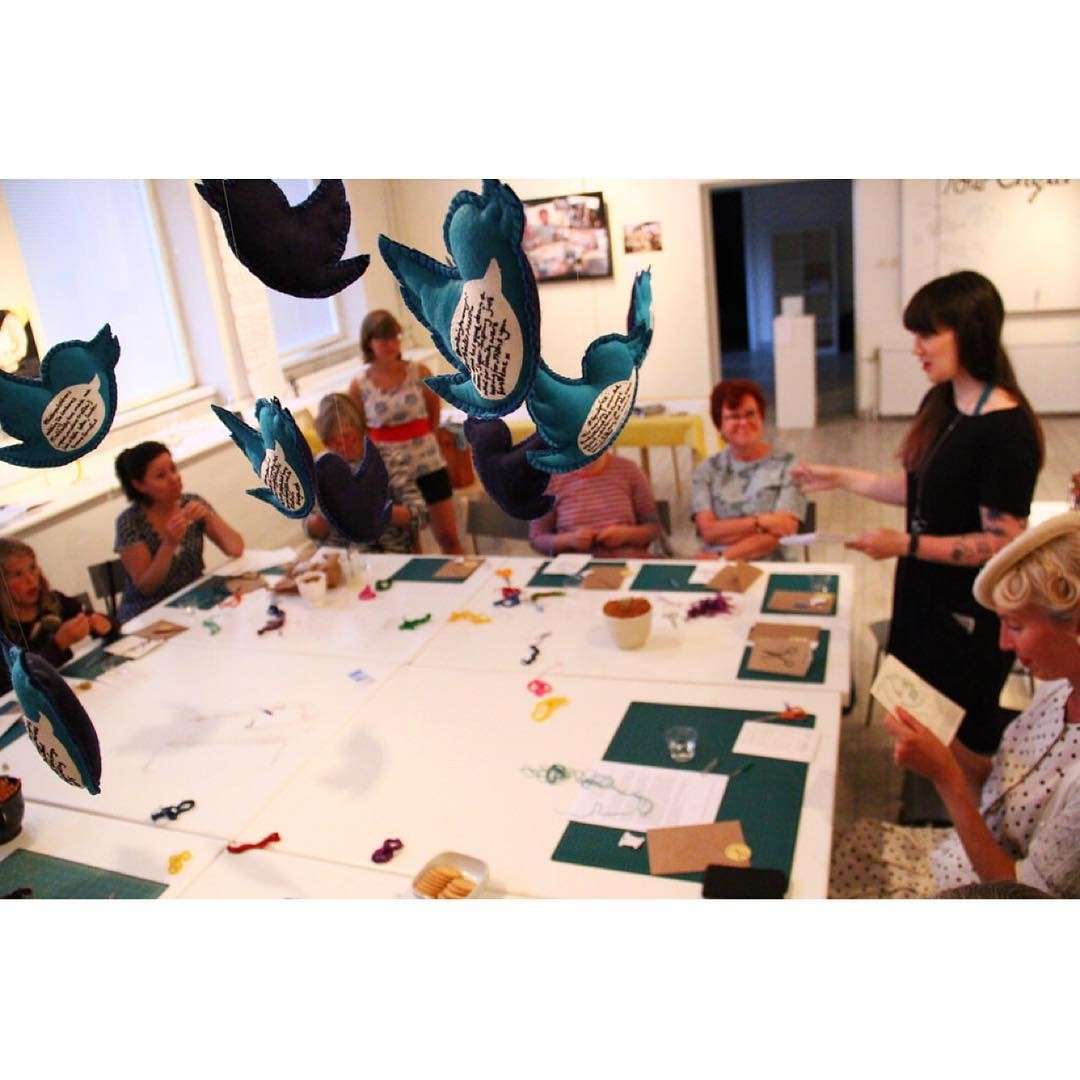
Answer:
[771,226,839,356]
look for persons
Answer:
[525,451,662,559]
[0,538,122,696]
[828,510,1080,900]
[791,270,1047,826]
[115,442,244,625]
[520,191,609,280]
[300,394,431,554]
[689,379,807,561]
[348,309,467,555]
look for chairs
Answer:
[87,558,125,619]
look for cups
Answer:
[296,570,328,607]
[665,723,698,762]
[603,596,653,652]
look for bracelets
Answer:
[907,534,918,559]
[718,551,727,562]
[753,512,760,534]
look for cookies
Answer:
[416,863,477,899]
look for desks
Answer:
[504,403,711,515]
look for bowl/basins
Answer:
[411,850,490,899]
[1,773,26,845]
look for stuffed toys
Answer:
[378,179,541,419]
[527,267,655,476]
[194,180,371,300]
[0,322,122,469]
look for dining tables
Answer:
[0,551,851,898]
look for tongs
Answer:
[779,526,864,547]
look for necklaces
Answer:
[911,380,982,532]
[151,501,179,517]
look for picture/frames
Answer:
[519,189,613,283]
